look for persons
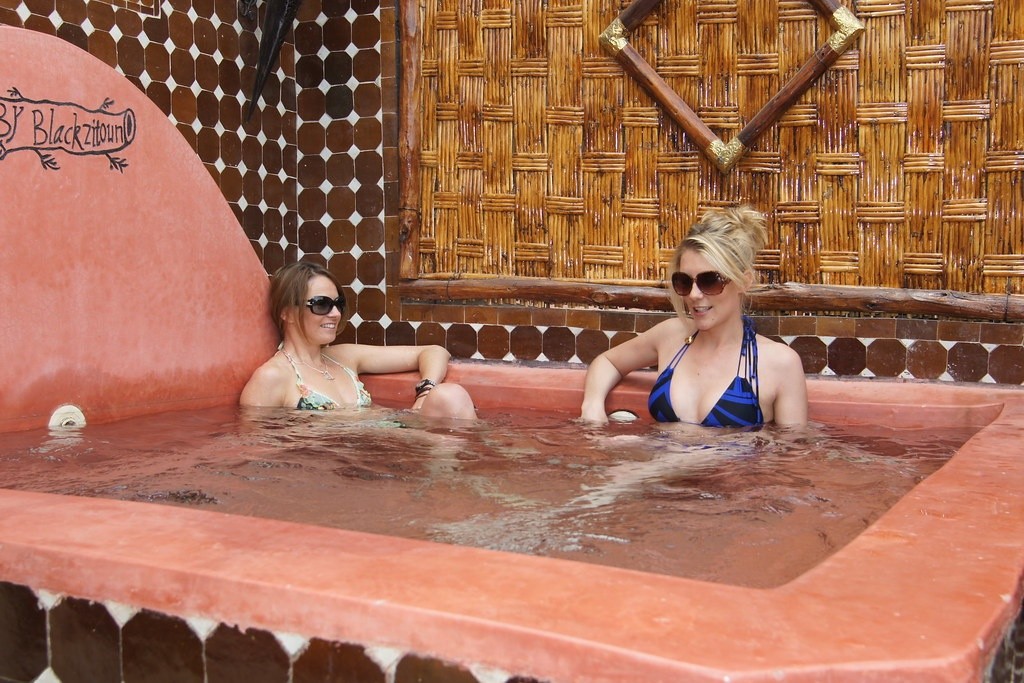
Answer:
[580,209,809,507]
[238,262,480,457]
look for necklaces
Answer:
[282,345,336,381]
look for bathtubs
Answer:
[1,362,1024,683]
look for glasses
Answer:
[671,270,746,296]
[293,296,345,315]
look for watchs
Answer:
[413,378,437,393]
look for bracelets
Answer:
[414,387,432,403]
